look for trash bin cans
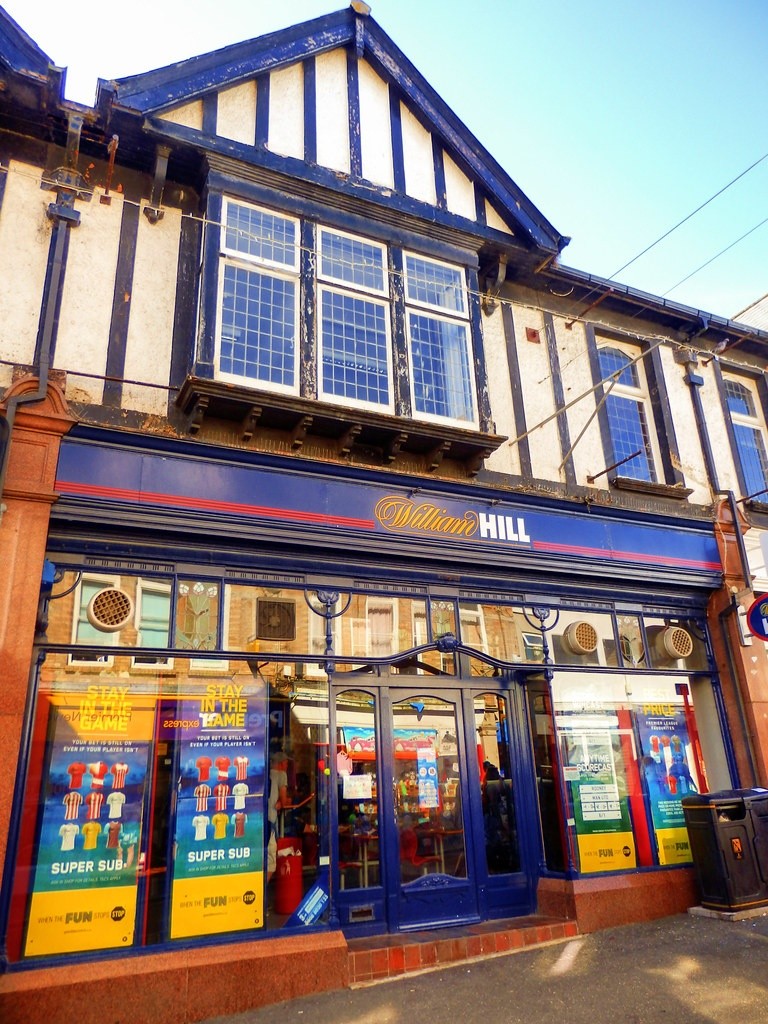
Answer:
[679,786,768,914]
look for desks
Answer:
[414,827,463,874]
[341,830,379,888]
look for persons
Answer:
[668,753,698,793]
[266,751,515,917]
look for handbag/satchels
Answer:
[498,796,508,814]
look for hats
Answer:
[272,752,293,761]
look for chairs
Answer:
[399,828,440,878]
[306,832,356,891]
